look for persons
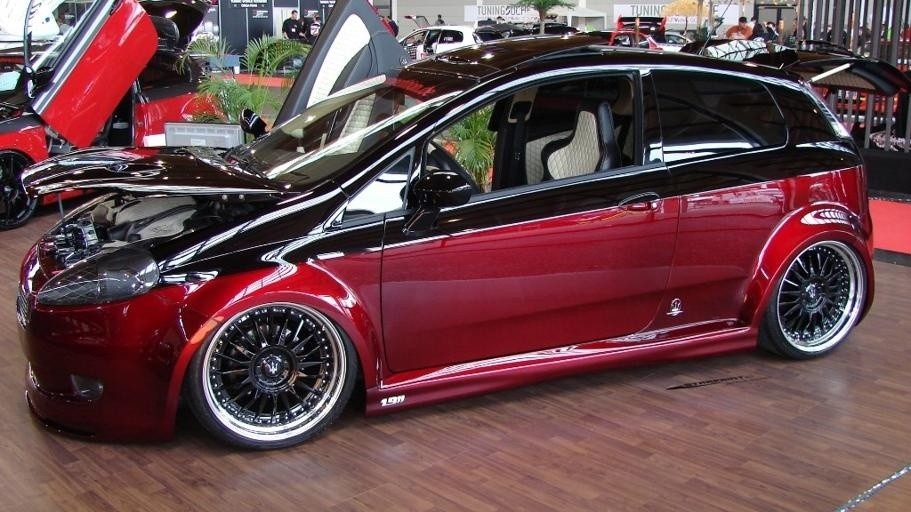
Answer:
[281,9,307,39]
[433,14,445,25]
[305,15,322,45]
[726,16,910,47]
[386,16,400,38]
[299,18,309,41]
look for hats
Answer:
[739,17,747,22]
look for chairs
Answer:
[526,101,634,190]
